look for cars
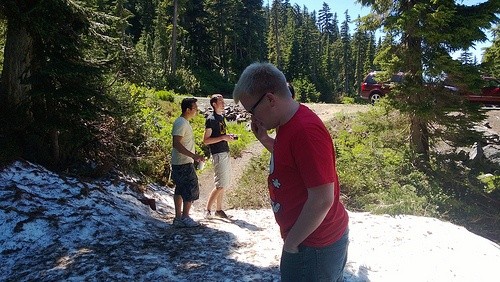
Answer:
[359,71,463,107]
[440,76,500,104]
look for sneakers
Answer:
[204,210,212,217]
[173,218,181,226]
[179,218,199,227]
[215,210,228,218]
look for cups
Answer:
[199,161,204,170]
[233,134,238,141]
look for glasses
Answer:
[245,92,275,118]
[189,105,197,110]
